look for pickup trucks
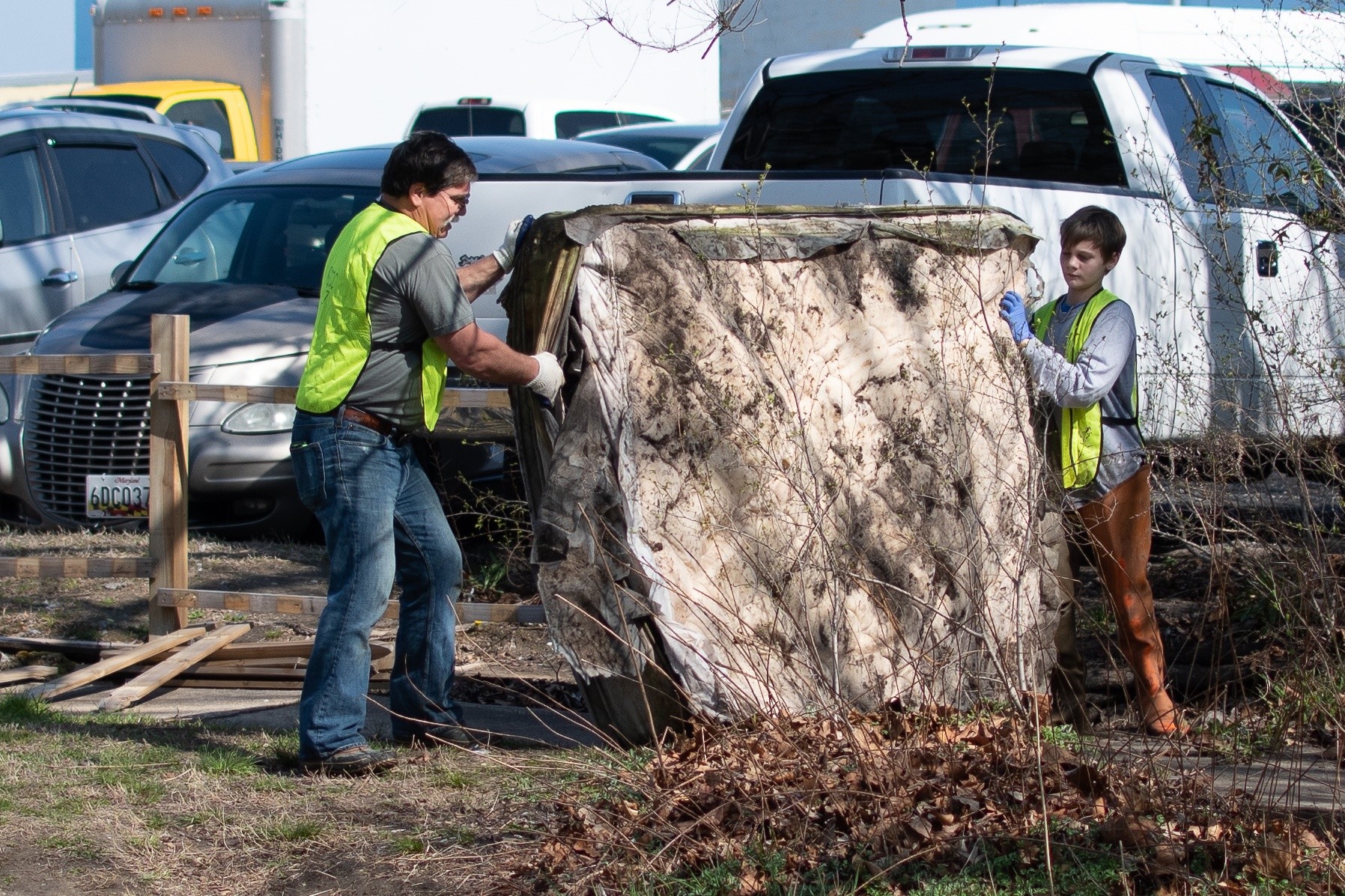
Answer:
[425,33,1345,464]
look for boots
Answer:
[1077,463,1190,735]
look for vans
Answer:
[2,3,1345,511]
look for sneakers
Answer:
[302,738,400,776]
[431,722,504,747]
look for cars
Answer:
[20,136,673,539]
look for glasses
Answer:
[443,189,468,210]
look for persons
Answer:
[999,205,1191,737]
[290,133,565,776]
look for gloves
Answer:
[523,350,565,405]
[999,290,1034,342]
[492,214,535,275]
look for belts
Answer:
[336,408,415,446]
